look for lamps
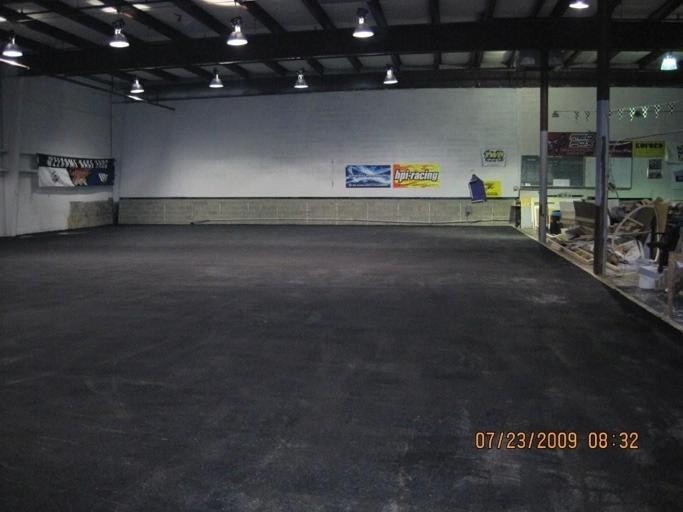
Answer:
[132,75,144,94]
[3,32,23,61]
[383,65,399,85]
[210,70,223,88]
[226,16,248,46]
[294,69,309,89]
[352,6,375,39]
[109,18,129,49]
[658,50,678,70]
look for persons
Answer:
[550,216,560,234]
